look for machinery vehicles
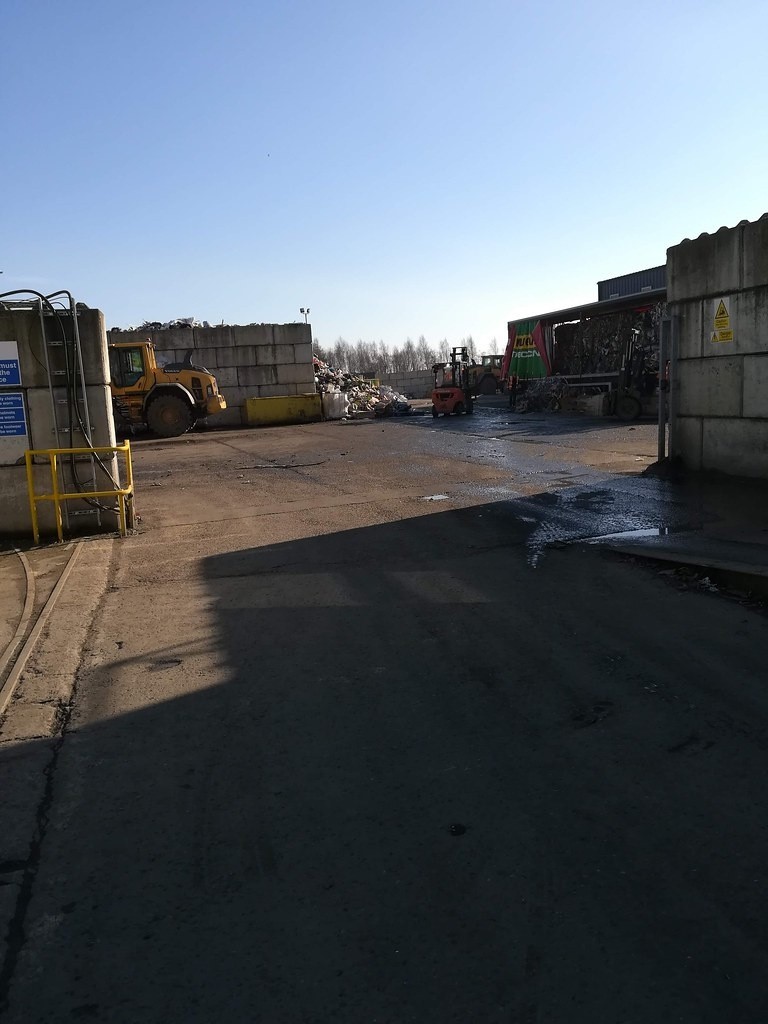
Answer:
[470,355,509,397]
[108,340,228,440]
[430,346,475,418]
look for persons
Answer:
[507,370,519,409]
